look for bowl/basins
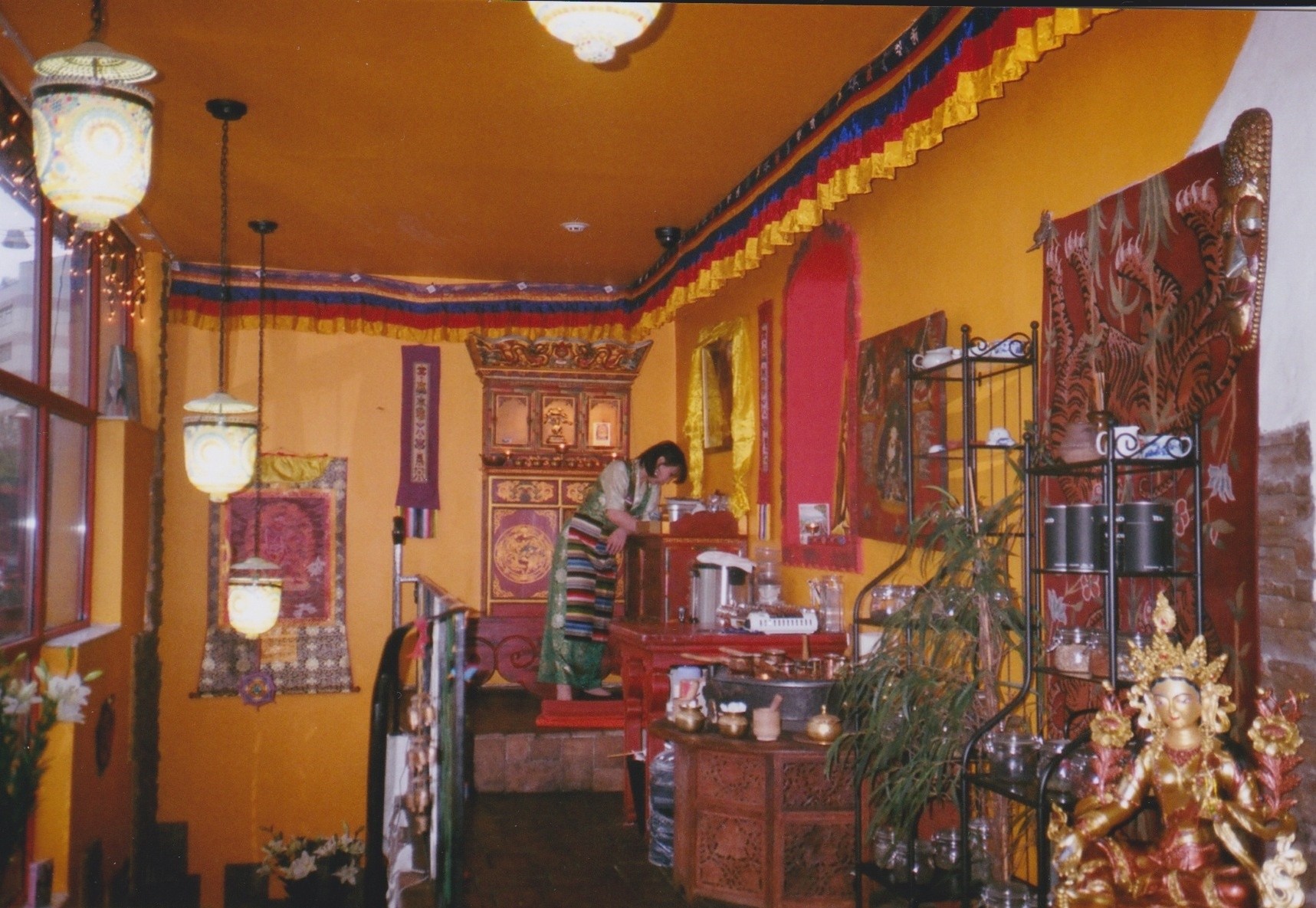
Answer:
[712,676,834,732]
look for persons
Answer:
[537,441,686,702]
[1050,633,1263,908]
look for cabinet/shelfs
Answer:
[608,533,749,626]
[649,677,850,908]
[851,320,1203,908]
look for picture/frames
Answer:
[700,335,734,454]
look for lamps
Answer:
[528,1,663,64]
[29,0,158,232]
[182,98,259,503]
[227,220,283,639]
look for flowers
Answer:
[256,822,365,887]
[0,645,103,873]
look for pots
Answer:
[692,564,747,630]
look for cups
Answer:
[912,339,1023,370]
[927,444,946,453]
[986,427,1014,447]
[1097,427,1193,460]
[672,698,698,724]
[1045,501,1166,571]
[753,708,780,741]
[731,649,846,681]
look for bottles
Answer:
[718,711,748,739]
[1046,627,1176,683]
[675,704,705,732]
[871,821,899,869]
[984,731,1041,784]
[647,740,675,868]
[870,585,959,623]
[888,839,928,888]
[934,829,964,869]
[980,880,1053,908]
[1072,752,1100,799]
[1037,739,1076,793]
[1058,422,1100,464]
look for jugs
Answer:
[808,575,844,633]
[666,665,709,722]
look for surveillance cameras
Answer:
[657,226,681,249]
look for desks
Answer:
[612,623,848,839]
